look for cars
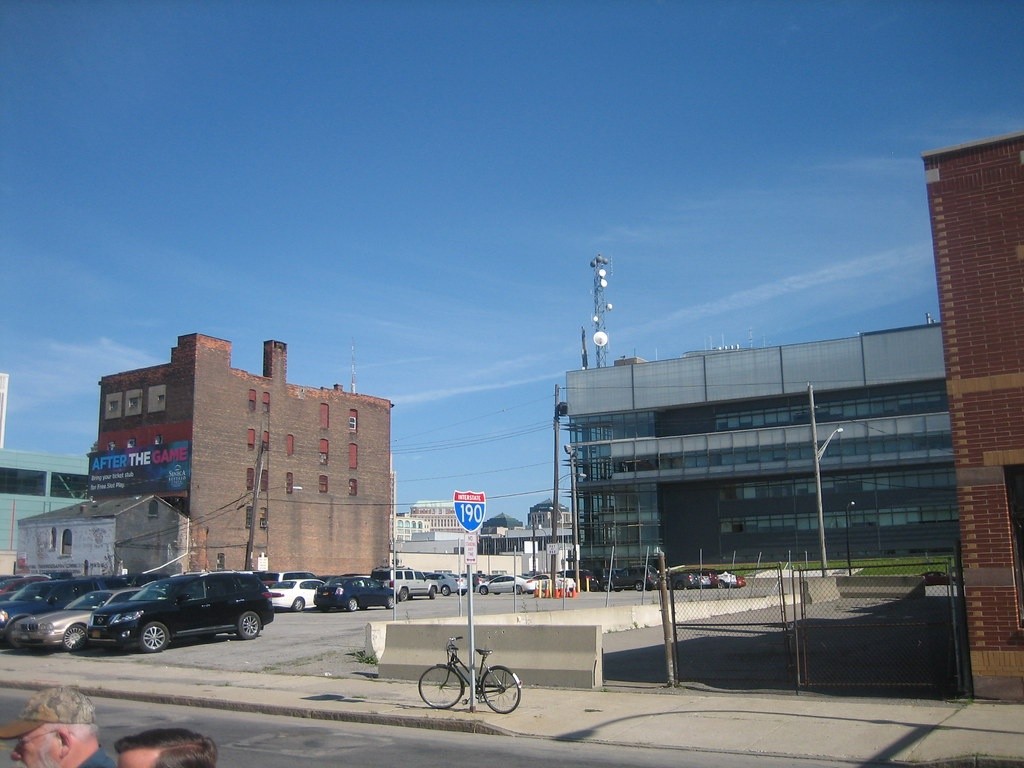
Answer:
[531,573,576,591]
[10,585,166,653]
[591,562,747,593]
[475,574,539,595]
[915,570,957,586]
[0,564,503,648]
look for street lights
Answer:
[549,506,564,569]
[564,445,587,592]
[550,473,587,593]
[813,428,845,571]
[243,484,304,571]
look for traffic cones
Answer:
[570,584,579,598]
[533,581,570,598]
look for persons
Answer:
[0,687,116,768]
[0,610,8,627]
[114,728,218,768]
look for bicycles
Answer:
[417,636,524,715]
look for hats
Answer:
[0,688,96,739]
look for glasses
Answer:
[18,730,57,749]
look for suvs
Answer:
[84,570,274,654]
[557,568,598,591]
